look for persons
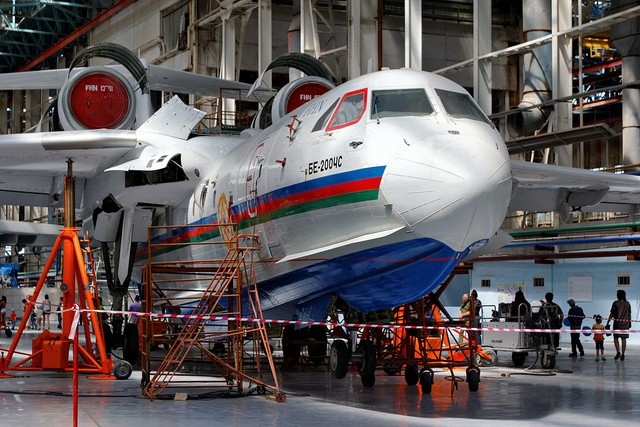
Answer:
[55,296,63,328]
[458,292,471,344]
[591,314,606,361]
[127,294,141,323]
[9,307,17,330]
[566,298,586,357]
[510,290,530,317]
[605,289,632,361]
[538,291,564,356]
[470,289,484,343]
[21,297,41,331]
[41,294,52,330]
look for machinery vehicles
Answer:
[0,41,639,391]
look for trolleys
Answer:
[475,300,561,368]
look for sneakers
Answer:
[568,352,577,357]
[601,356,608,360]
[614,352,621,360]
[579,352,585,356]
[620,355,625,361]
[595,358,599,361]
